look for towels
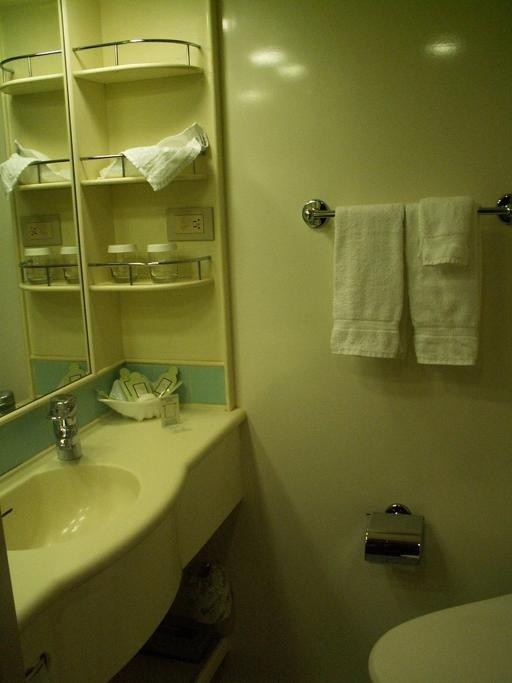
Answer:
[330,203,408,357]
[406,202,484,367]
[419,196,475,268]
[98,119,208,192]
[0,140,70,196]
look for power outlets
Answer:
[166,207,213,242]
[19,214,61,248]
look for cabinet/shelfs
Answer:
[61,0,227,292]
[0,50,82,292]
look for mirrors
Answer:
[0,0,95,426]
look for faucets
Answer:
[0,389,16,414]
[45,394,82,460]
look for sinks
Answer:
[4,455,142,558]
